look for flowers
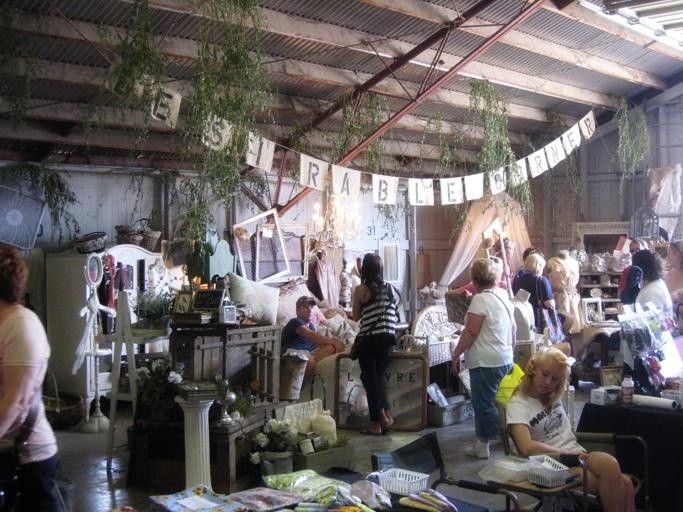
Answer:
[250,419,297,465]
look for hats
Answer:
[296,296,316,308]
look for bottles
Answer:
[621,375,634,407]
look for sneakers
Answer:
[464,446,490,459]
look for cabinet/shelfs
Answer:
[47,243,190,424]
[94,292,167,470]
[576,272,622,327]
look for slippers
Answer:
[359,428,382,435]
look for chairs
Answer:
[373,400,651,512]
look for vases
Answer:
[260,451,293,475]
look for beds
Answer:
[223,272,466,431]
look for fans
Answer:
[0,185,47,250]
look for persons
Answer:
[616,239,682,378]
[0,243,68,512]
[550,247,581,332]
[504,341,636,512]
[350,253,400,435]
[281,296,345,398]
[449,233,556,459]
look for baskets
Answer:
[115,218,161,250]
[528,454,570,489]
[379,469,430,498]
[75,231,107,254]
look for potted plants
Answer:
[294,436,352,472]
[129,288,173,336]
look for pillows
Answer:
[228,272,280,325]
[277,277,326,323]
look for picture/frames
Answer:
[598,365,624,387]
[582,298,602,325]
[173,290,195,314]
[219,305,236,324]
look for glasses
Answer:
[630,248,640,253]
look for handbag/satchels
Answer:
[0,448,29,511]
[540,308,565,345]
[351,331,373,360]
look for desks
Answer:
[174,321,281,402]
[574,378,682,512]
[137,466,490,512]
